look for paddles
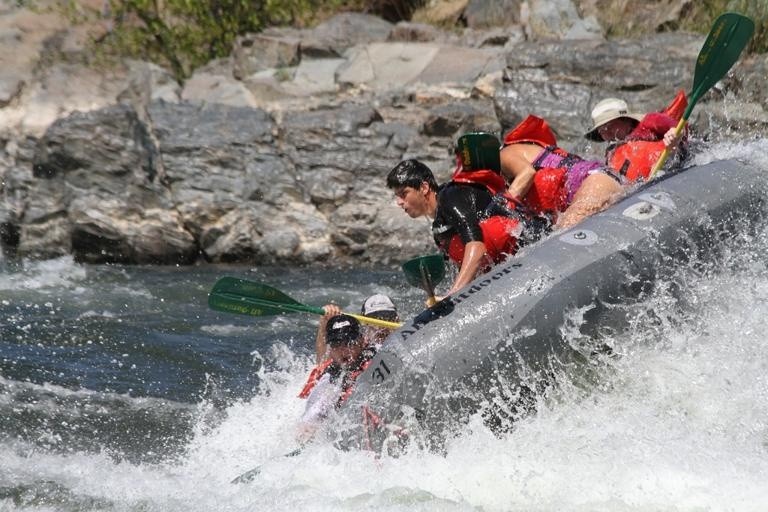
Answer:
[459,131,501,177]
[206,278,402,331]
[402,256,446,306]
[649,13,755,182]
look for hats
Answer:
[362,294,398,316]
[583,97,640,143]
[325,315,362,345]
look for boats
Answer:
[315,141,766,457]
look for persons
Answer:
[452,115,625,236]
[385,159,554,296]
[297,314,377,421]
[313,292,400,365]
[584,95,694,176]
